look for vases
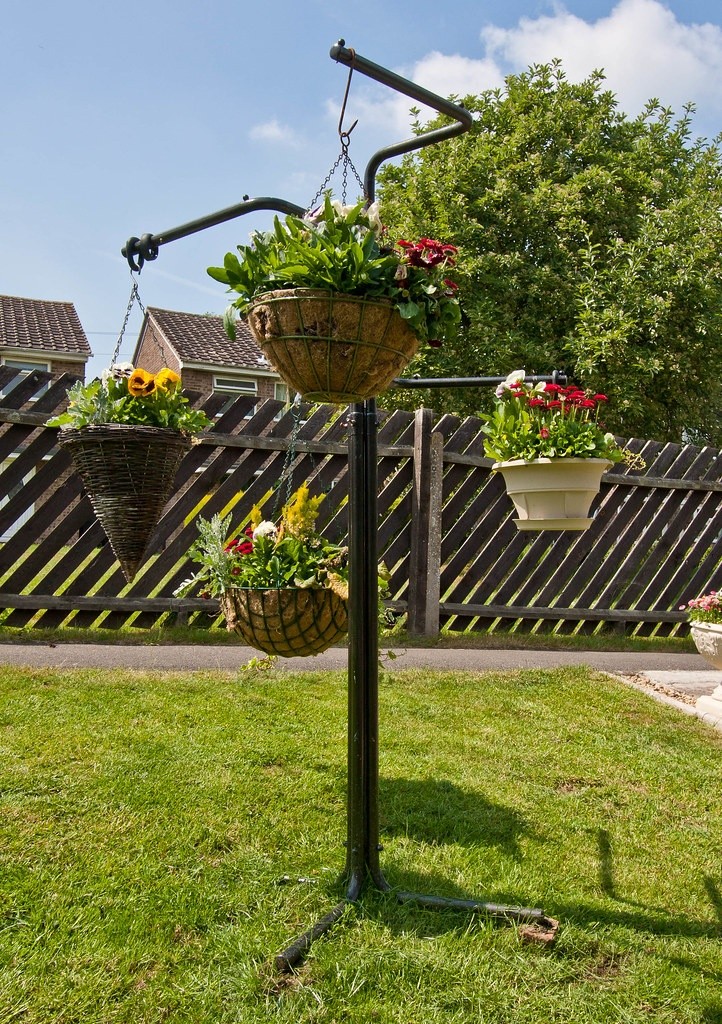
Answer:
[240,292,423,406]
[690,620,722,701]
[491,456,615,530]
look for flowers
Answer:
[475,370,645,472]
[43,365,214,440]
[679,588,722,623]
[169,486,393,608]
[207,187,463,350]
